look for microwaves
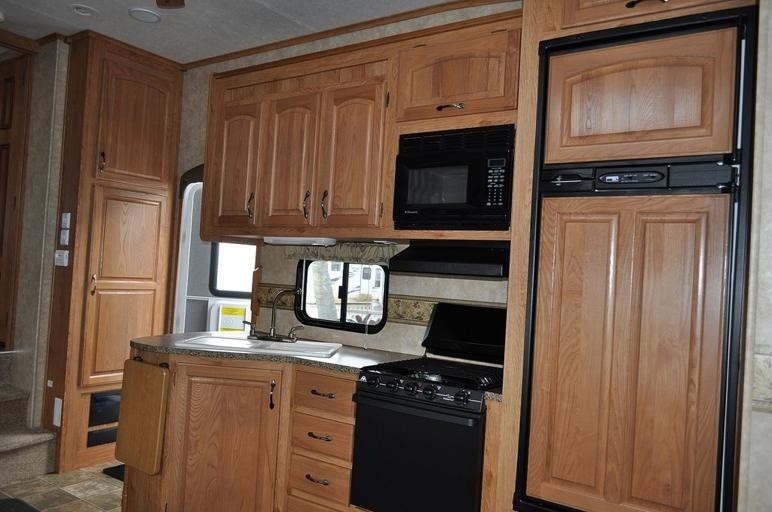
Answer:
[392,123,516,231]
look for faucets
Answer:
[269,288,302,336]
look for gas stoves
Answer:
[360,355,504,394]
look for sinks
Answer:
[178,336,260,351]
[265,340,341,357]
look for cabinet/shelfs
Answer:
[65,28,185,189]
[393,11,521,123]
[169,359,282,512]
[551,0,750,28]
[38,182,176,481]
[200,63,268,242]
[525,192,744,512]
[270,37,393,239]
[287,366,355,512]
[536,24,743,165]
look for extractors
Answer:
[389,239,511,281]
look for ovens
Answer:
[350,393,486,511]
